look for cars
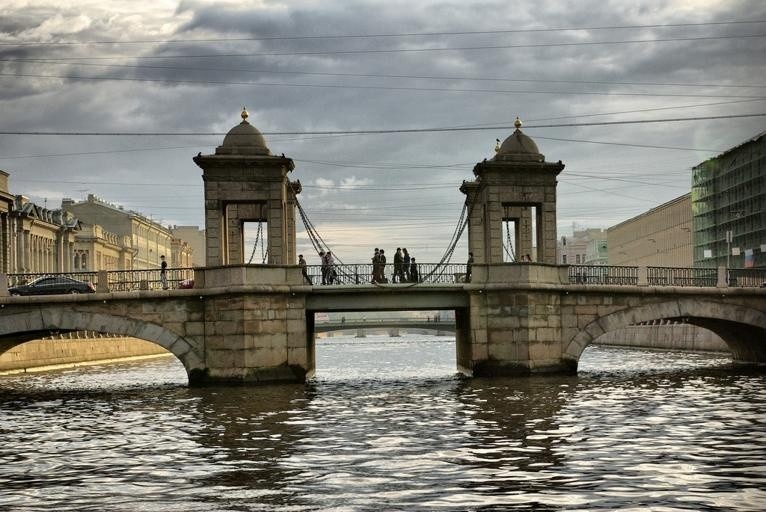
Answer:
[178,278,194,288]
[8,276,96,297]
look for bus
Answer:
[314,312,329,324]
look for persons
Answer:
[527,253,532,261]
[298,255,314,285]
[319,248,418,284]
[466,252,474,281]
[159,255,169,289]
[576,274,583,282]
[521,255,527,261]
